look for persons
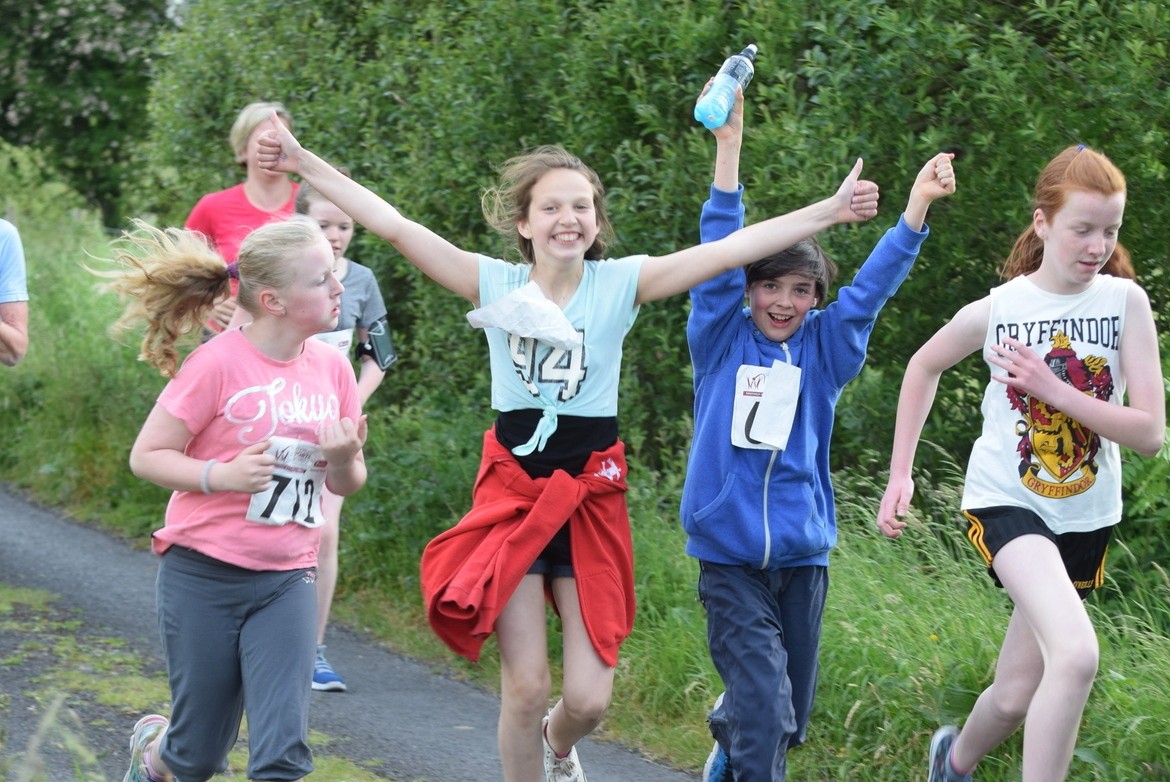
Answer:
[257,110,877,782]
[228,167,397,690]
[102,216,369,782]
[878,145,1164,782]
[681,77,957,782]
[0,218,27,366]
[185,102,301,344]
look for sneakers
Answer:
[541,710,592,782]
[309,645,354,692]
[126,715,169,781]
[703,691,742,782]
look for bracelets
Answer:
[200,460,216,494]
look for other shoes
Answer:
[925,722,971,782]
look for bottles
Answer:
[694,44,757,129]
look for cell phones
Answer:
[371,320,397,370]
